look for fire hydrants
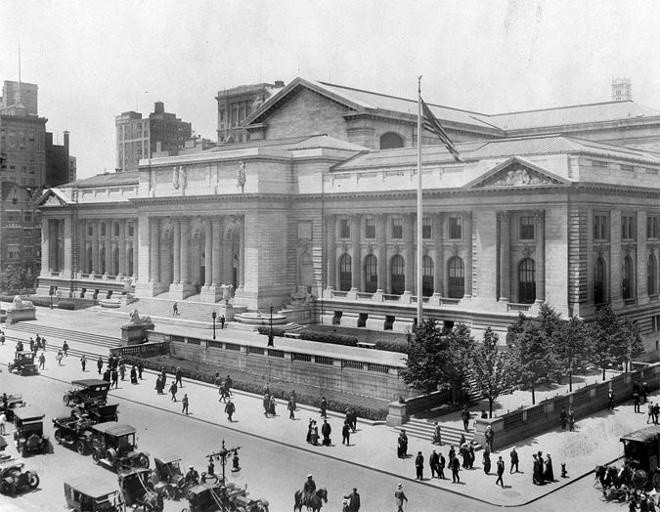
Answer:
[560,461,568,477]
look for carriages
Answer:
[592,426,660,503]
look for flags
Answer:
[420,96,463,161]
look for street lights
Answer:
[267,301,278,347]
[211,310,218,339]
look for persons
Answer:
[173,302,177,315]
[221,315,225,328]
